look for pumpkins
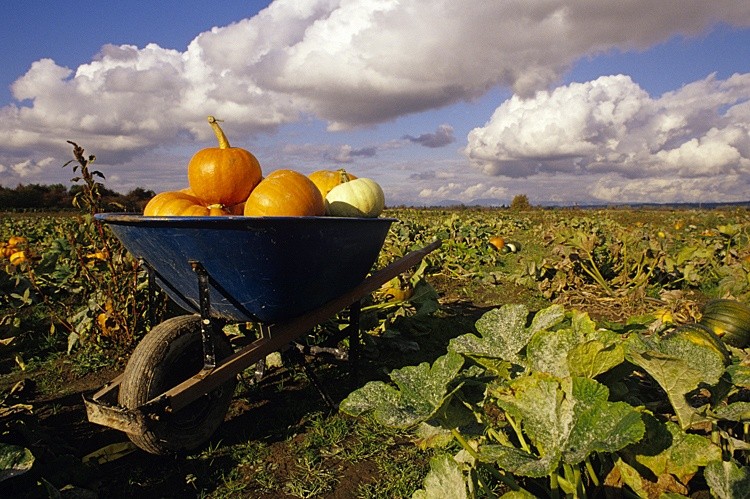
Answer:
[1,235,750,363]
[143,118,385,217]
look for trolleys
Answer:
[80,210,442,457]
